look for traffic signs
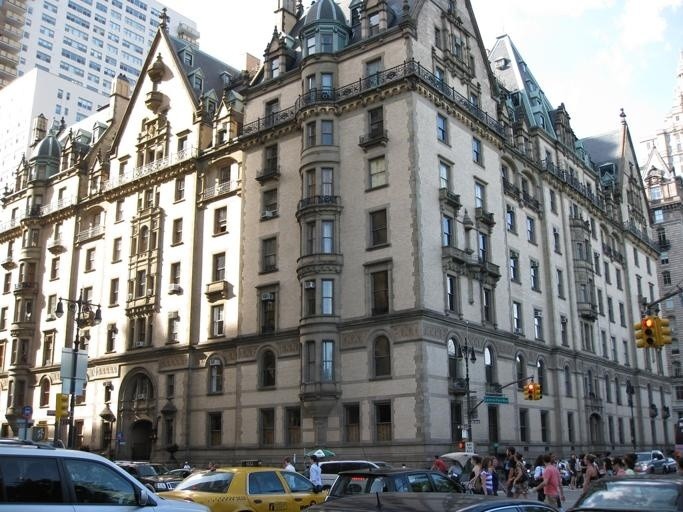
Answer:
[456,424,469,430]
[482,395,509,406]
[470,420,480,424]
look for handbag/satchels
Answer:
[528,476,544,487]
[513,468,529,485]
[474,474,482,493]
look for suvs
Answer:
[0,436,209,512]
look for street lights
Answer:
[455,336,475,453]
[54,287,103,448]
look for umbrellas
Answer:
[304,448,336,458]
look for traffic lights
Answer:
[655,316,673,347]
[457,442,465,450]
[534,384,543,401]
[641,315,658,349]
[55,394,69,419]
[523,384,528,401]
[527,384,535,401]
[631,319,644,350]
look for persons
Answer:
[285,458,296,488]
[309,455,321,486]
[429,448,637,512]
[183,462,191,469]
[209,461,215,488]
